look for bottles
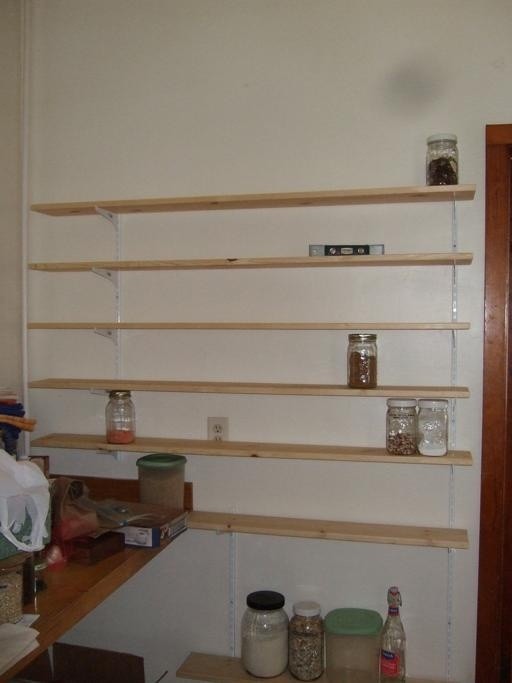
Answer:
[103,391,135,446]
[0,544,48,626]
[239,590,324,681]
[384,397,450,458]
[322,607,383,683]
[135,452,187,512]
[380,586,406,683]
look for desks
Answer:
[0,475,193,682]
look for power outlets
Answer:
[207,416,229,441]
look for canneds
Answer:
[106,392,134,445]
[387,399,418,456]
[419,400,449,456]
